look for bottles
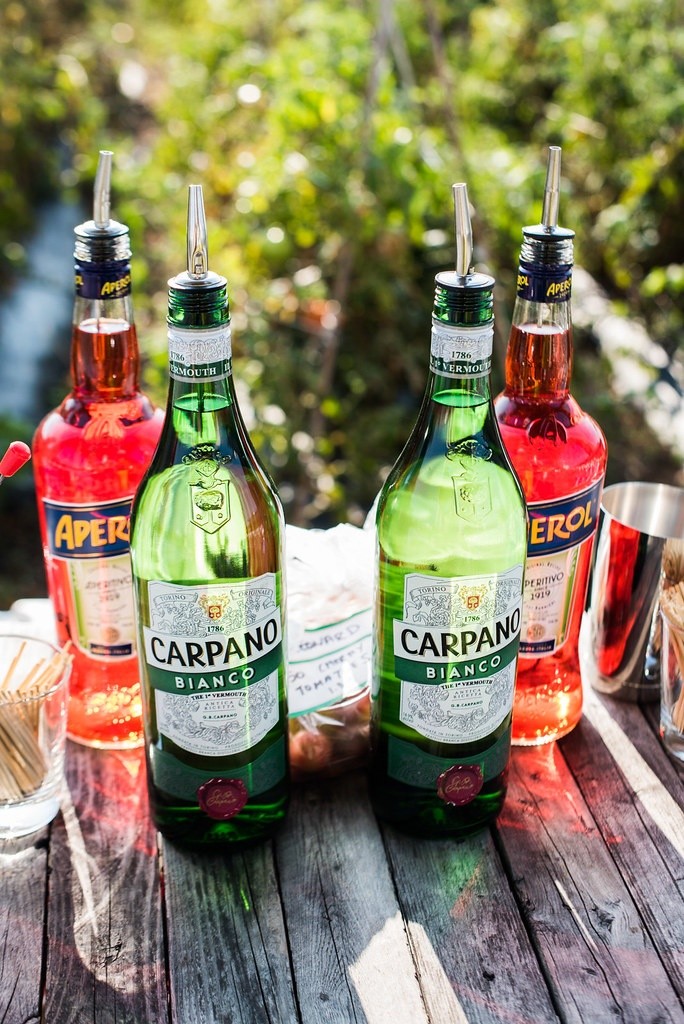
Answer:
[29,147,166,751]
[372,185,531,841]
[126,184,294,857]
[488,142,609,748]
[259,276,387,801]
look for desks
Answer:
[0,608,684,1024]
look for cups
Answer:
[0,633,74,839]
[657,538,684,763]
[585,480,684,705]
